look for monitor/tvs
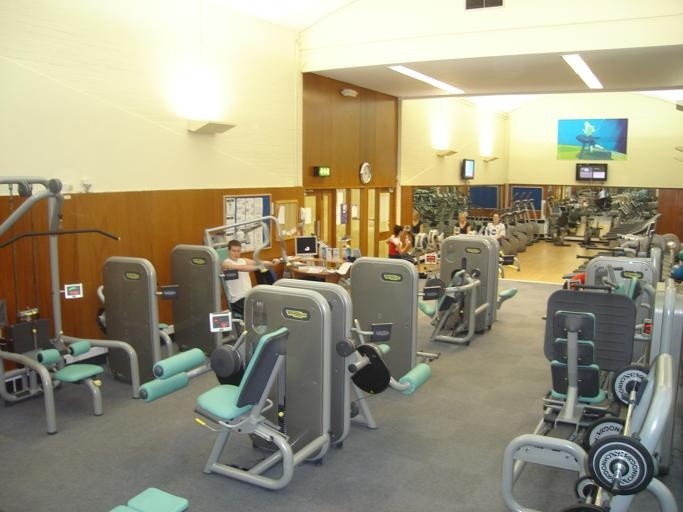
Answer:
[462,159,474,179]
[576,163,608,181]
[295,236,318,256]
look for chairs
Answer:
[547,308,607,426]
[193,324,297,491]
[418,270,467,328]
[217,246,246,321]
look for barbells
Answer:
[588,364,654,495]
[562,416,625,512]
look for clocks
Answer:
[358,161,372,185]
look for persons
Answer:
[583,122,603,153]
[547,196,559,212]
[384,225,418,263]
[455,213,471,234]
[412,211,423,234]
[222,240,302,314]
[579,193,588,207]
[665,241,683,281]
[484,212,506,248]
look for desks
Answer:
[289,255,345,285]
